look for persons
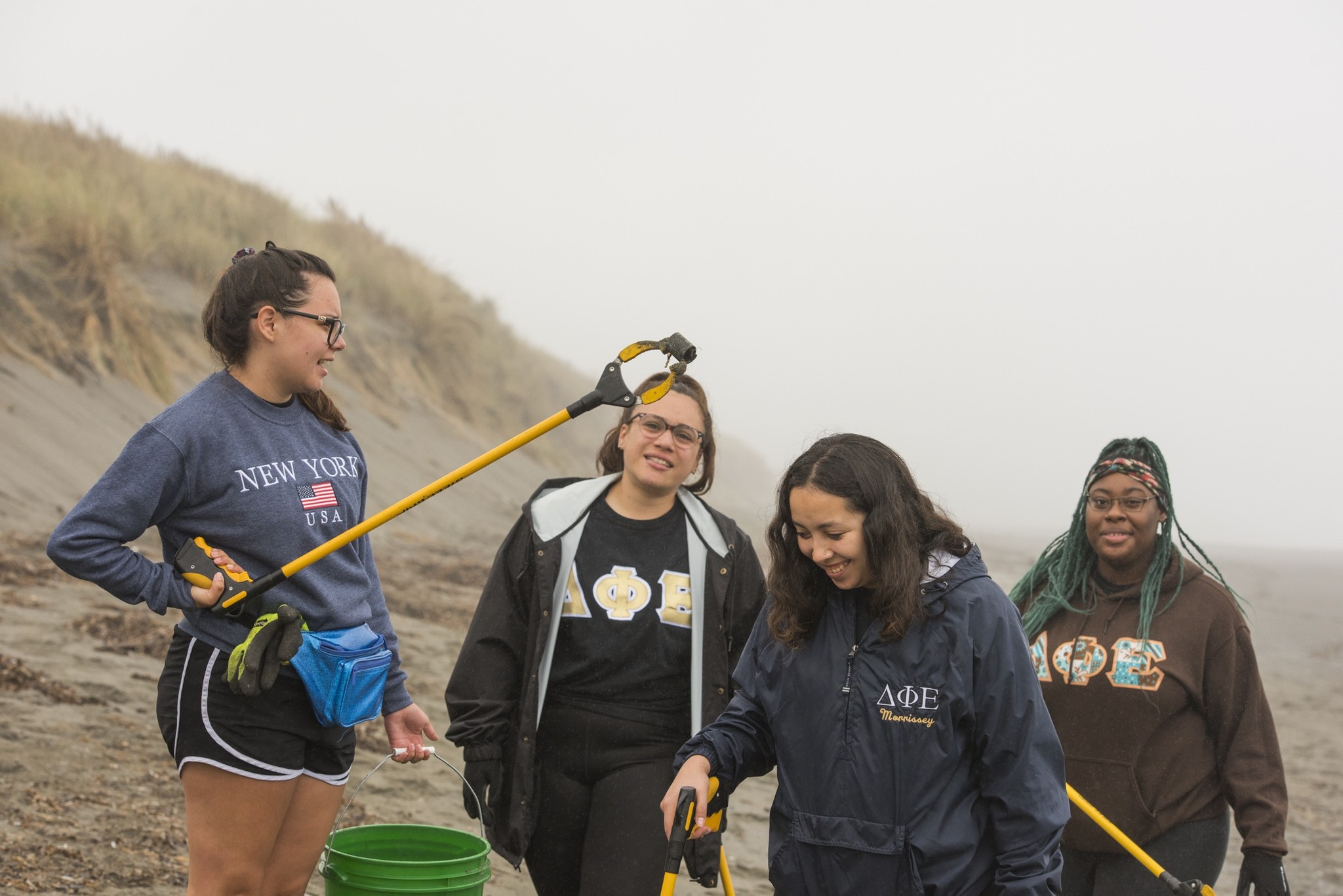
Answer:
[1008,434,1293,896]
[661,432,1070,896]
[46,245,441,895]
[444,371,767,896]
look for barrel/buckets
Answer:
[317,746,493,896]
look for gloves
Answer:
[462,760,505,827]
[221,602,309,700]
[1237,850,1292,896]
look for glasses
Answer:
[620,412,704,449]
[251,306,348,344]
[1086,491,1156,513]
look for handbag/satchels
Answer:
[177,606,393,727]
